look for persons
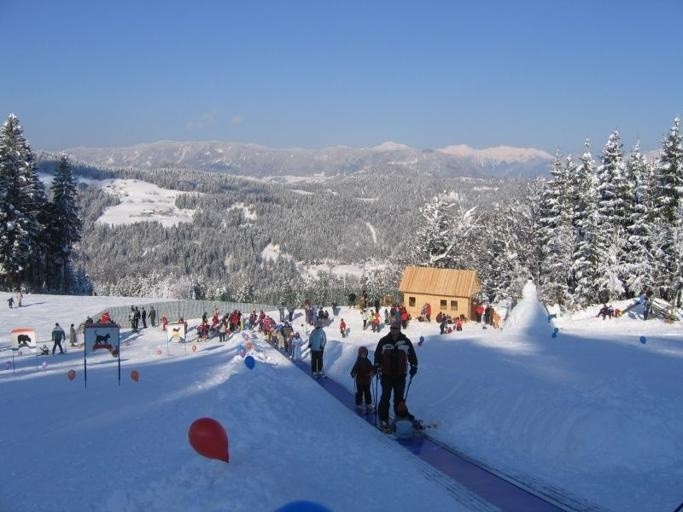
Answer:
[332,300,337,315]
[68,322,78,348]
[308,307,329,326]
[306,321,326,378]
[277,301,296,322]
[274,322,303,363]
[16,290,23,308]
[172,318,188,343]
[350,346,375,412]
[49,322,66,356]
[348,290,409,333]
[196,308,278,344]
[594,303,607,320]
[373,319,418,430]
[6,296,15,308]
[83,312,112,323]
[421,303,462,334]
[473,303,494,326]
[606,304,615,320]
[340,319,345,337]
[128,306,168,334]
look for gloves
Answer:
[409,365,418,376]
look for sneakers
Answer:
[356,404,374,410]
[313,371,326,378]
[380,420,390,429]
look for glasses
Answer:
[390,326,399,329]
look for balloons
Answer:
[552,328,559,333]
[187,417,229,465]
[41,360,48,370]
[551,333,556,338]
[246,342,251,350]
[639,335,646,344]
[244,356,255,370]
[157,349,161,356]
[129,369,140,383]
[15,350,22,358]
[270,499,334,512]
[4,361,11,371]
[236,345,243,353]
[192,345,196,352]
[66,370,76,382]
[239,347,246,360]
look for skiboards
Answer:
[313,373,328,381]
[371,413,426,442]
[354,404,377,416]
